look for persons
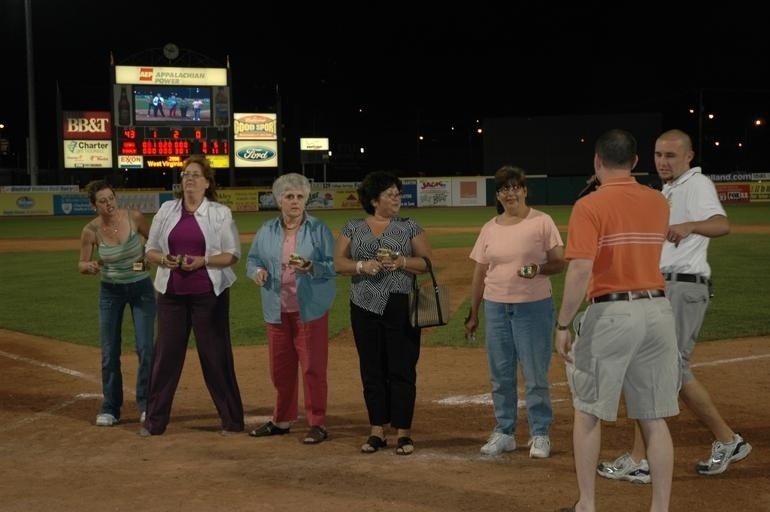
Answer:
[552,133,677,512]
[591,125,752,482]
[335,172,434,456]
[79,179,157,426]
[145,92,205,121]
[140,156,248,436]
[245,172,340,444]
[462,165,566,460]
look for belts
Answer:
[592,290,664,302]
[662,273,709,285]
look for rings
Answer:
[372,267,376,273]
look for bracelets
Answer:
[160,255,165,266]
[355,259,363,274]
[202,254,210,268]
[402,256,407,273]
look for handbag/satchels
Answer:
[409,289,447,328]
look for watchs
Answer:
[554,318,569,332]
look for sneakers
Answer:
[698,434,752,476]
[530,435,550,459]
[94,411,146,426]
[597,453,650,483]
[479,432,516,453]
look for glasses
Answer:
[496,185,523,194]
[180,170,206,177]
[386,192,402,200]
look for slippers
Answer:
[395,436,414,456]
[303,425,326,444]
[250,421,291,436]
[362,435,387,452]
